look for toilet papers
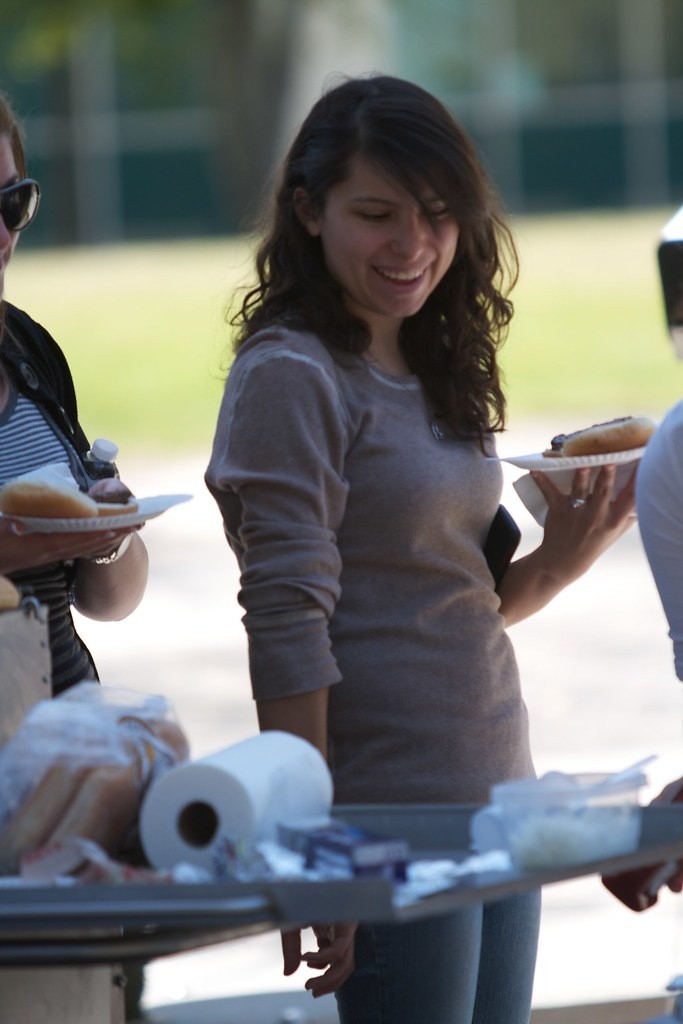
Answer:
[138,730,333,870]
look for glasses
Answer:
[0,179,43,236]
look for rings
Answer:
[569,496,588,508]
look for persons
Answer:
[205,78,644,1024]
[601,206,683,910]
[0,99,152,1023]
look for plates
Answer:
[482,445,648,471]
[0,495,192,531]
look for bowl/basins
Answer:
[489,770,646,870]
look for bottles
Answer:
[78,438,120,492]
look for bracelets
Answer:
[79,531,136,565]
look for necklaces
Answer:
[366,347,444,442]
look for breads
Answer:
[0,482,99,519]
[97,503,139,517]
[0,714,189,876]
[561,417,654,455]
